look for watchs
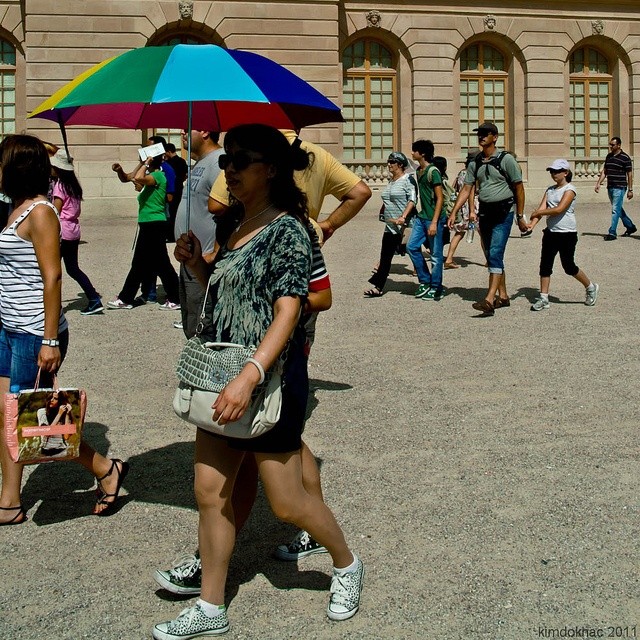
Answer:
[515,213,522,219]
[40,338,60,348]
[143,163,151,168]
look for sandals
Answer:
[443,262,459,268]
[1,505,26,525]
[493,296,510,309]
[473,300,495,315]
[93,457,129,515]
[363,287,384,295]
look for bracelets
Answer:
[399,215,407,220]
[242,358,265,385]
[628,189,633,192]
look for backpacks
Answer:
[429,165,463,223]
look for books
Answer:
[137,143,167,162]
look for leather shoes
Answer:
[604,234,615,240]
[624,227,637,236]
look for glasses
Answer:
[477,132,492,137]
[387,159,396,164]
[218,152,276,173]
[608,142,619,146]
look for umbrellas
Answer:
[24,45,347,248]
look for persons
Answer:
[447,122,528,315]
[364,153,416,297]
[111,132,175,304]
[208,123,371,250]
[48,152,104,315]
[173,127,225,341]
[522,158,599,312]
[107,137,183,311]
[444,148,489,270]
[407,140,446,300]
[433,156,451,270]
[152,124,367,640]
[173,320,184,329]
[596,138,637,241]
[152,215,332,596]
[0,134,131,527]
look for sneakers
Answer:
[422,286,444,300]
[80,301,104,314]
[585,283,599,305]
[107,298,133,309]
[159,299,181,310]
[327,558,364,621]
[531,298,550,311]
[153,553,229,594]
[173,321,183,329]
[153,601,229,640]
[415,283,430,297]
[276,531,328,559]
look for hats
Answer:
[389,152,408,167]
[472,121,498,134]
[545,159,571,172]
[50,149,74,172]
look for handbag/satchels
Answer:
[172,334,284,440]
[4,363,86,464]
